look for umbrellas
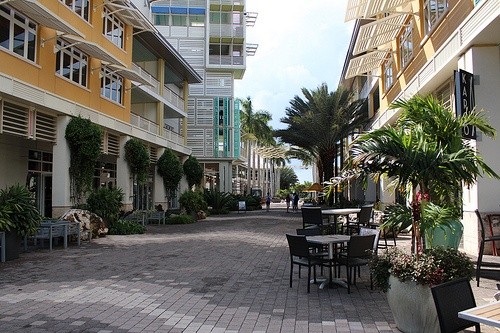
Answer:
[302,183,324,202]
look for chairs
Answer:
[286,201,400,294]
[430,276,480,333]
[474,209,500,287]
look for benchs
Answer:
[127,211,165,227]
[21,218,80,252]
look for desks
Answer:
[322,209,361,235]
[305,235,351,289]
[457,300,500,328]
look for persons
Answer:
[292,193,299,214]
[286,194,291,213]
[265,193,271,211]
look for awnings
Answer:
[344,0,416,79]
[0,0,157,90]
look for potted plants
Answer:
[0,183,42,261]
[425,200,464,249]
[384,247,476,333]
